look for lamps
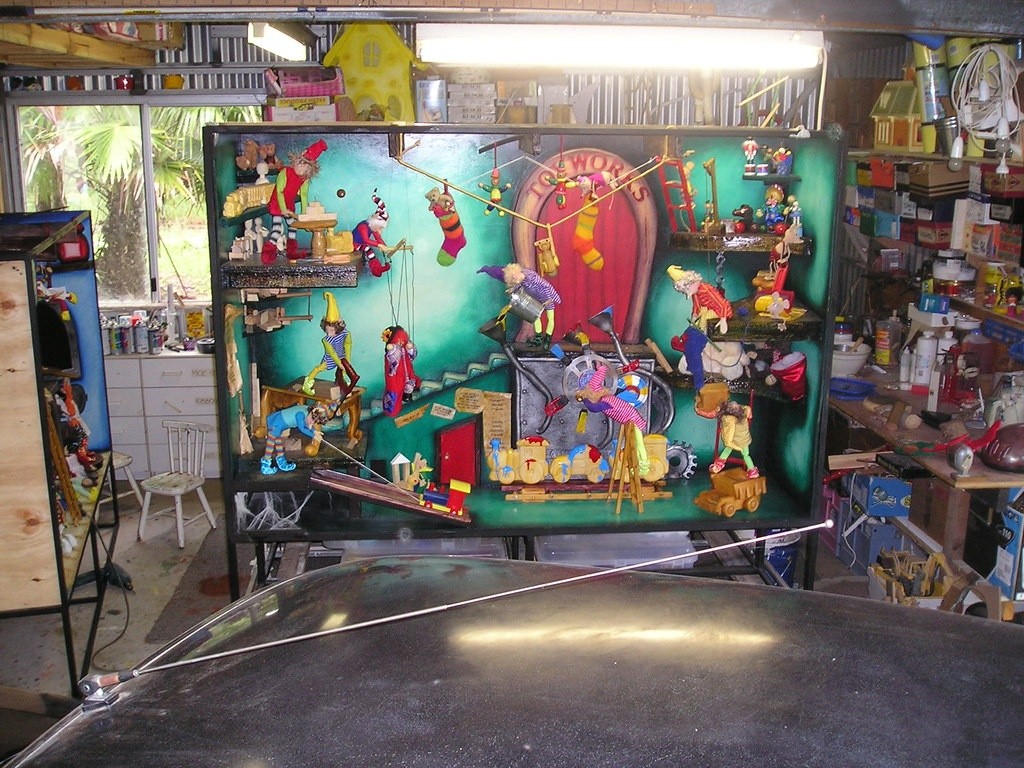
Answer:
[247,21,316,62]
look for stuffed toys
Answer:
[678,342,750,380]
[732,205,757,229]
[545,164,579,209]
[757,185,796,233]
[235,140,259,169]
[770,223,804,275]
[260,144,281,169]
[425,188,466,267]
[478,169,511,217]
[743,137,758,163]
[746,342,779,385]
[768,292,789,331]
[476,262,561,350]
[675,161,696,208]
[534,240,560,278]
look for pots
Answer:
[114,76,134,91]
[162,74,186,91]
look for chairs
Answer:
[137,420,218,548]
[91,449,144,523]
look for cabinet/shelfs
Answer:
[216,249,371,522]
[104,347,222,480]
[666,174,823,395]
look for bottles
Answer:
[898,346,910,382]
[937,331,959,365]
[101,315,164,356]
[914,331,938,385]
[940,356,959,401]
[960,329,995,398]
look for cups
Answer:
[549,103,573,123]
[497,104,539,125]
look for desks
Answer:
[829,352,1024,490]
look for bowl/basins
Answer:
[830,341,872,379]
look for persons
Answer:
[789,201,801,225]
[576,366,650,475]
[667,266,733,333]
[254,218,268,253]
[694,383,762,478]
[261,405,327,473]
[256,163,269,185]
[352,189,391,278]
[303,291,354,397]
[244,220,257,254]
[577,171,618,199]
[261,140,328,262]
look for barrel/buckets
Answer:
[834,323,853,342]
[734,527,801,588]
[508,286,548,325]
[934,115,960,158]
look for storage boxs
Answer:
[130,21,185,51]
[841,147,1024,332]
[414,79,539,123]
[818,466,1024,624]
